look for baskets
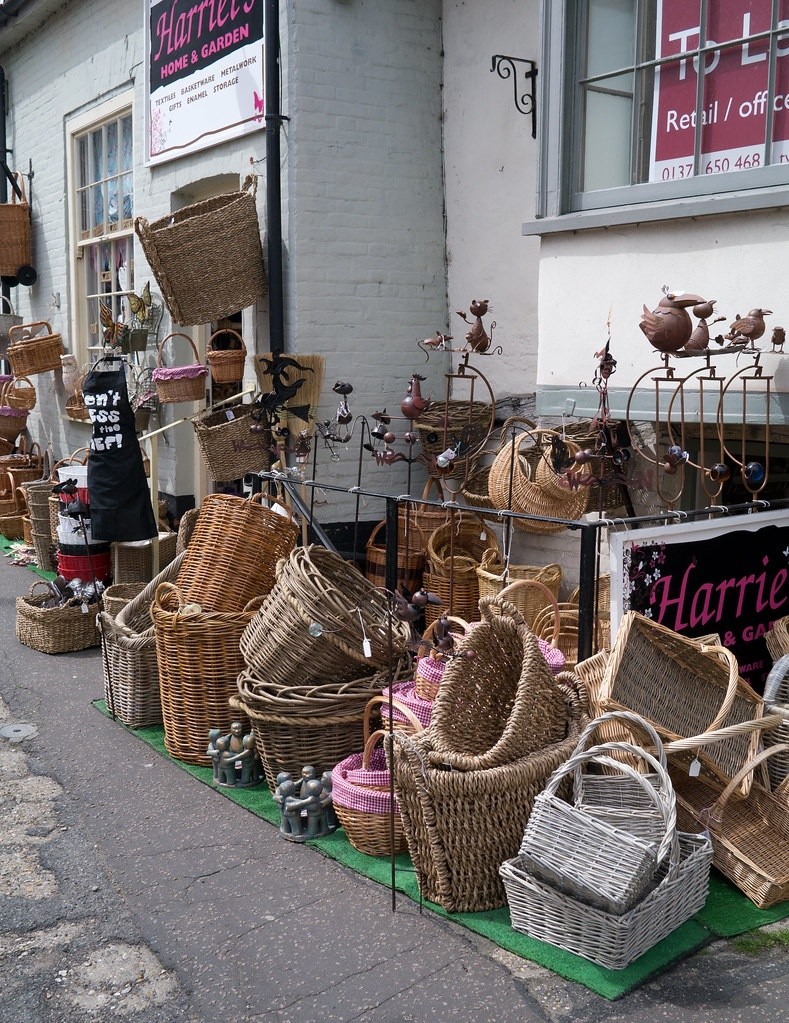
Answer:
[6,322,65,378]
[0,295,24,359]
[152,332,207,404]
[206,328,247,383]
[133,173,268,327]
[0,170,30,278]
[0,376,789,972]
